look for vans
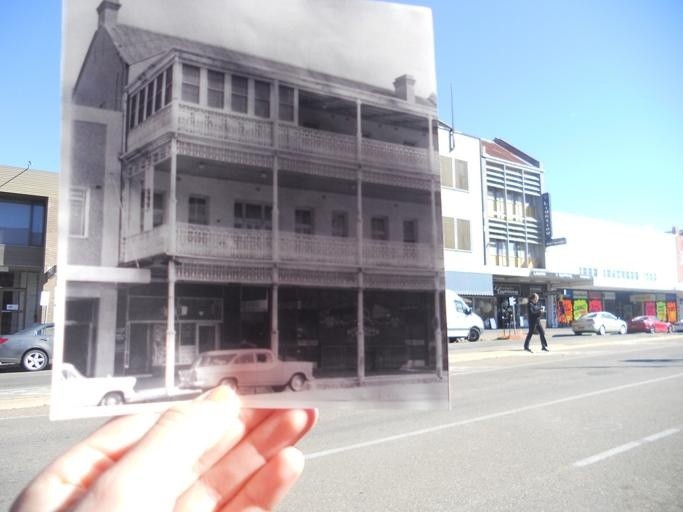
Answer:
[446,288,484,342]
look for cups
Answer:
[541,306,545,313]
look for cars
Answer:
[0,322,54,371]
[571,312,683,335]
[62,348,314,406]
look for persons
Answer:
[559,302,567,324]
[523,292,550,353]
[10,386,318,512]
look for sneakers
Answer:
[541,345,549,351]
[523,345,534,353]
